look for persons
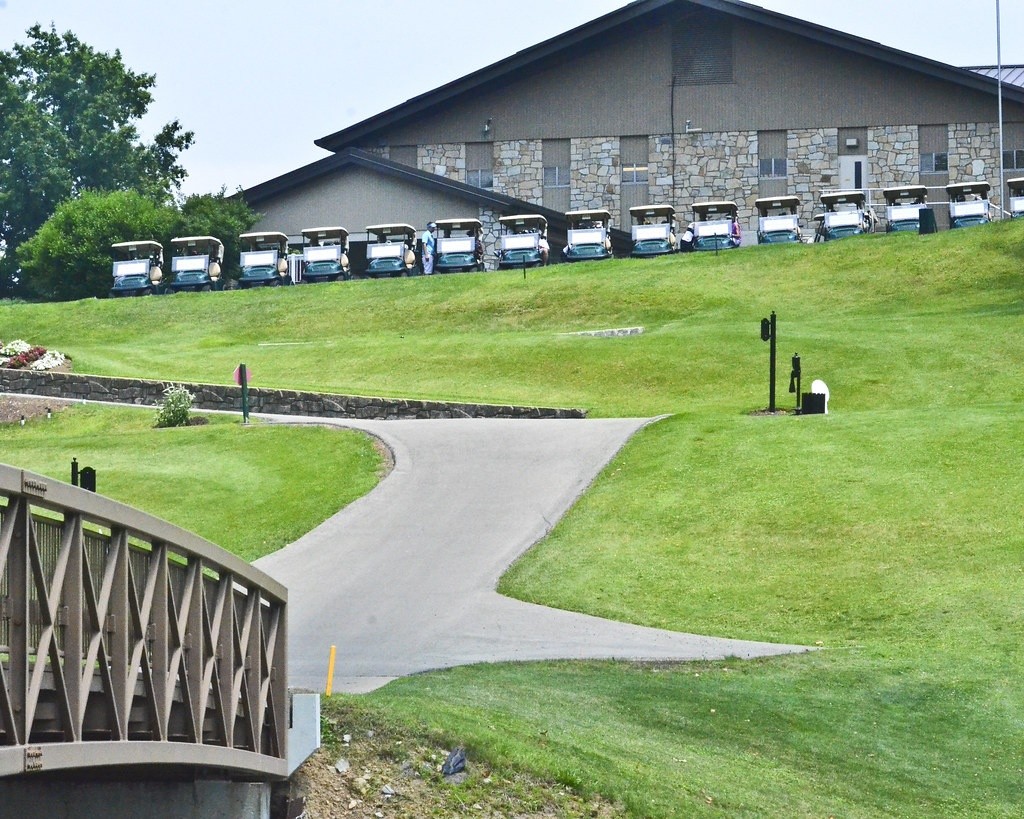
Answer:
[421,221,438,274]
[536,229,550,266]
[680,222,696,251]
[726,215,741,245]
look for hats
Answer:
[426,222,437,228]
